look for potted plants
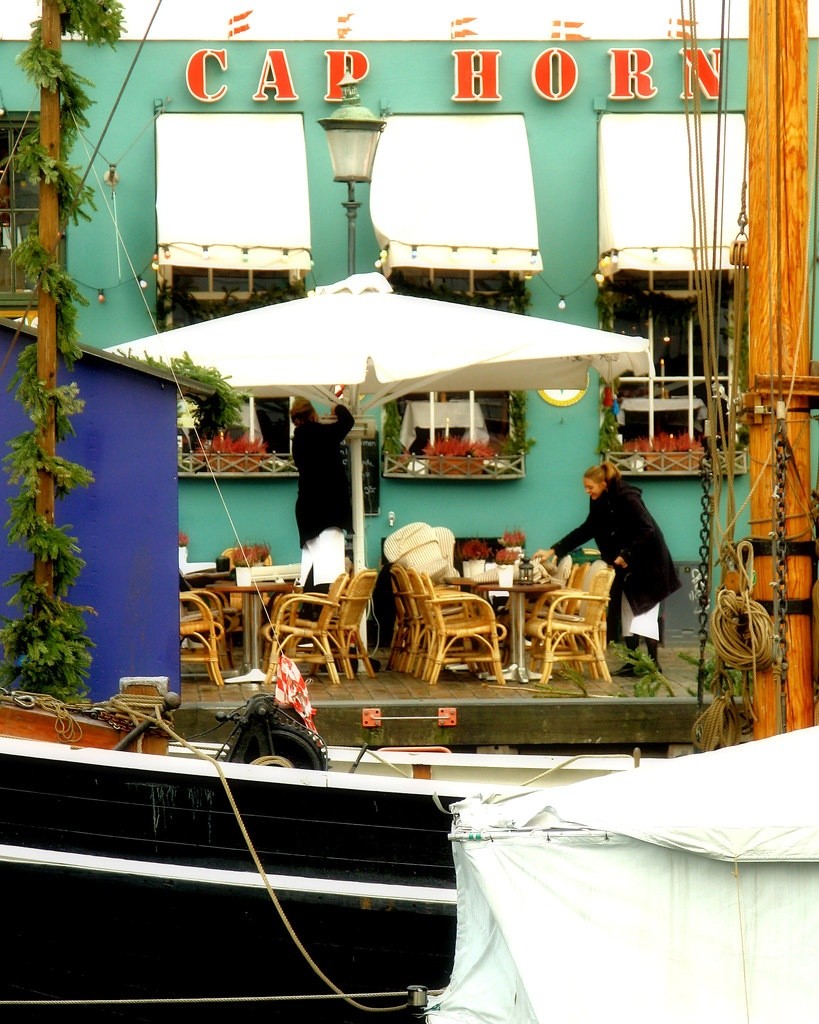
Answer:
[457,542,471,578]
[622,431,704,471]
[178,528,188,569]
[422,435,494,475]
[192,430,269,472]
[252,545,271,580]
[485,547,497,574]
[466,539,489,578]
[498,528,526,581]
[495,549,519,587]
[230,546,253,588]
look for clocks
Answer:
[537,372,589,406]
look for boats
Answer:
[0,686,819,1023]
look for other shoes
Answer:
[609,663,662,678]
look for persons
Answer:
[532,459,684,678]
[289,400,356,645]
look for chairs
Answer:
[261,565,378,683]
[180,585,235,688]
[384,565,506,685]
[497,554,618,684]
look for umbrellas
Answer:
[105,269,654,679]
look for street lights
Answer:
[316,69,386,278]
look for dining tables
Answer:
[211,581,298,685]
[477,577,563,684]
